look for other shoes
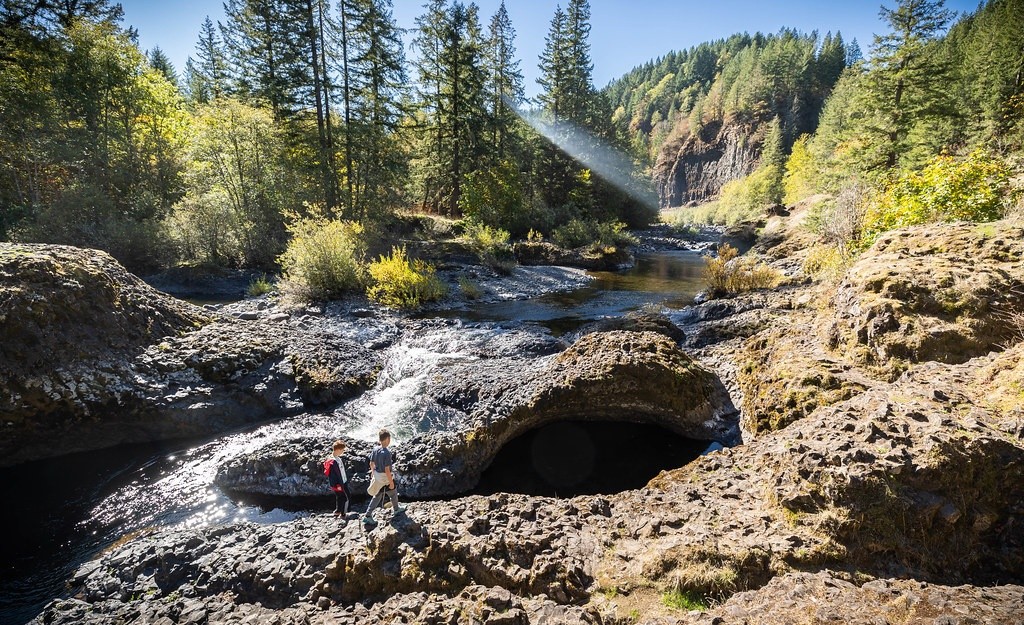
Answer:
[333,511,339,516]
[340,514,349,519]
[362,514,378,526]
[390,505,407,518]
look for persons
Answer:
[324,440,350,520]
[362,429,407,525]
